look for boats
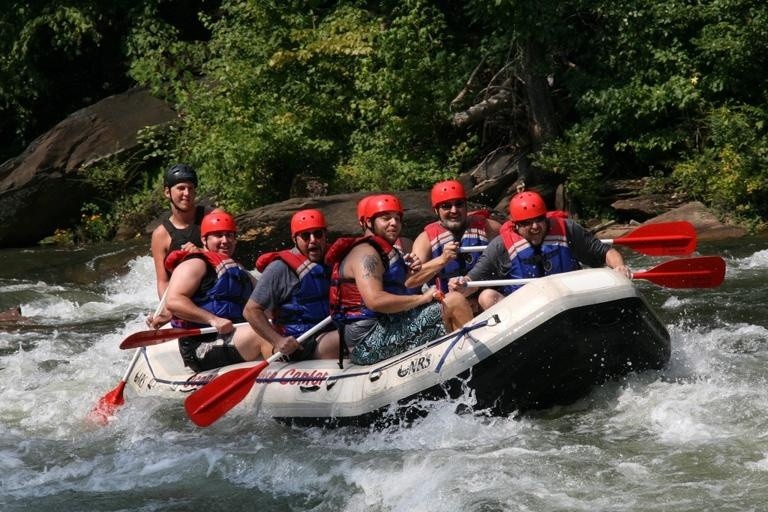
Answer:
[126,265,674,438]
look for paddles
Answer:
[119,319,272,349]
[83,286,169,427]
[455,221,698,256]
[461,256,726,288]
[185,315,333,427]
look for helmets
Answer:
[431,180,466,209]
[200,208,237,245]
[366,194,403,221]
[290,208,327,238]
[510,191,546,222]
[357,194,378,221]
[164,164,198,189]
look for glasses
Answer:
[439,200,466,210]
[298,229,325,241]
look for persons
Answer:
[144,164,213,330]
[164,212,282,373]
[328,194,473,367]
[243,209,354,360]
[448,191,631,311]
[405,180,502,318]
[357,196,413,256]
